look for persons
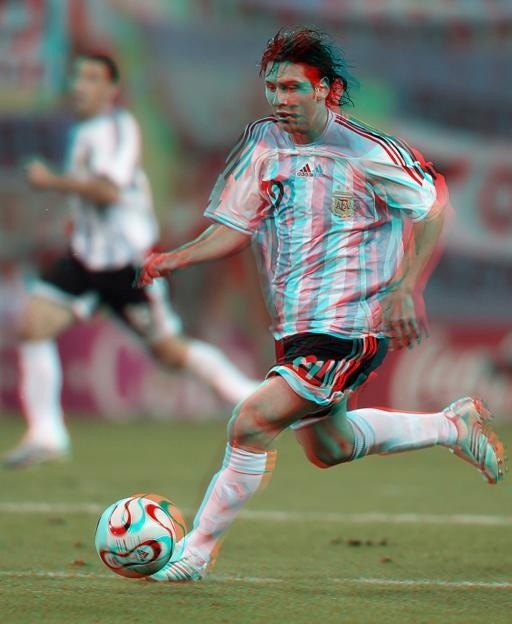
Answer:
[131,25,507,581]
[3,53,260,467]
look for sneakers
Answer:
[145,537,204,583]
[442,395,504,487]
[4,428,73,466]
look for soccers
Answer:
[95,498,176,578]
